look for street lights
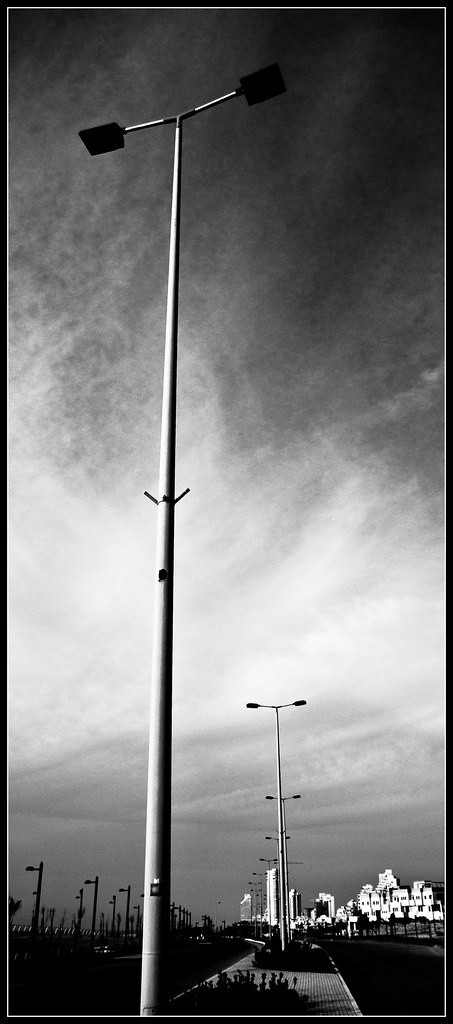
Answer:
[76,62,290,1015]
[23,861,195,923]
[246,699,306,954]
